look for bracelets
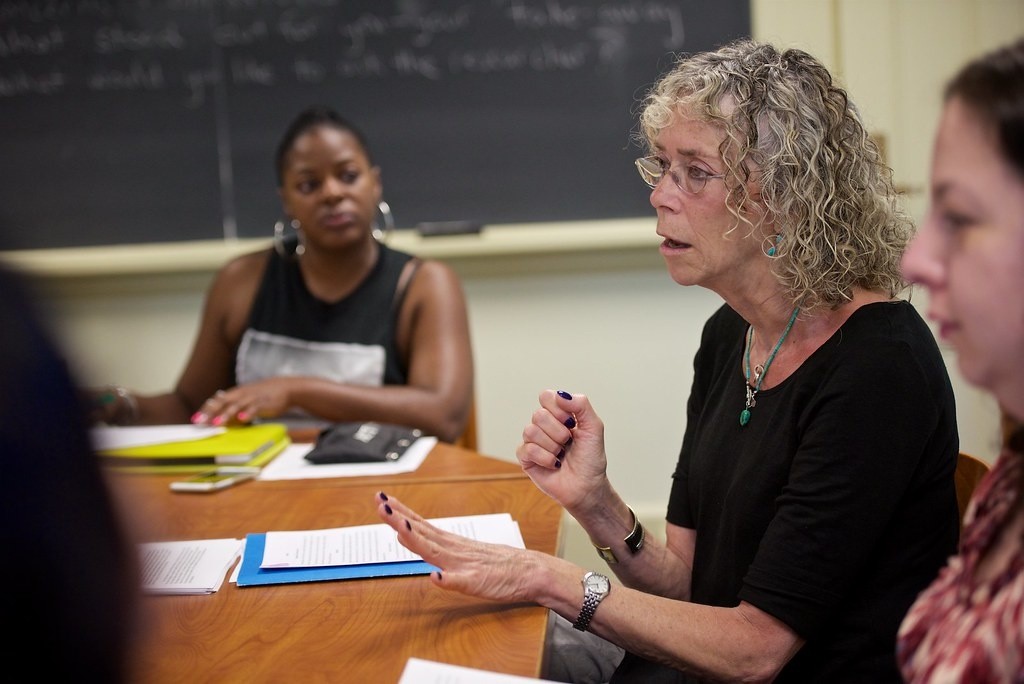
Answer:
[117,384,141,425]
[591,504,645,564]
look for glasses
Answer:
[634,155,763,195]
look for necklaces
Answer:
[740,294,806,426]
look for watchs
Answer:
[573,571,611,632]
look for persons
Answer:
[0,262,141,684]
[375,43,959,684]
[78,109,474,444]
[901,37,1024,684]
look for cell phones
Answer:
[170,466,260,492]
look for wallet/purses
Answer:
[303,420,421,463]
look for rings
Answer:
[214,390,227,406]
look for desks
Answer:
[100,421,563,684]
